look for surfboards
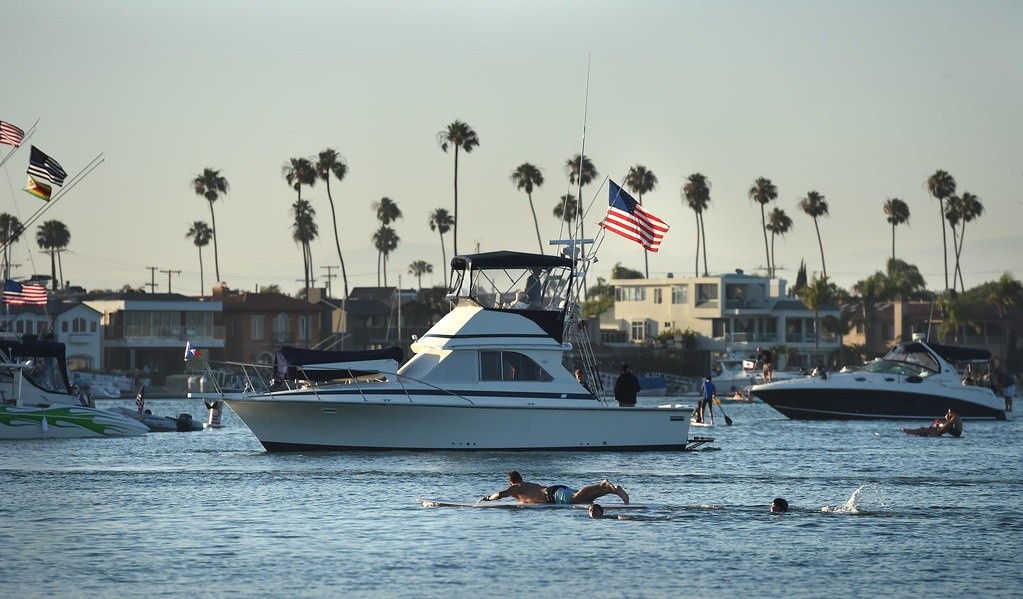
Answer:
[873,432,965,439]
[415,496,667,510]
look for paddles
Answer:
[712,395,732,425]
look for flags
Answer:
[24,174,52,202]
[1,279,48,307]
[0,121,24,148]
[135,384,145,412]
[598,178,670,252]
[27,146,68,187]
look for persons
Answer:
[575,369,590,394]
[588,504,604,519]
[71,372,94,408]
[962,367,1016,412]
[245,384,251,393]
[690,401,702,423]
[481,471,629,505]
[700,375,716,425]
[904,408,963,437]
[615,364,641,407]
[511,291,532,310]
[525,268,542,309]
[770,497,789,512]
[797,364,826,378]
[755,347,772,384]
[733,390,748,400]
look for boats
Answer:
[701,347,803,405]
[183,52,717,457]
[0,334,204,437]
[746,298,1009,423]
[634,376,670,398]
